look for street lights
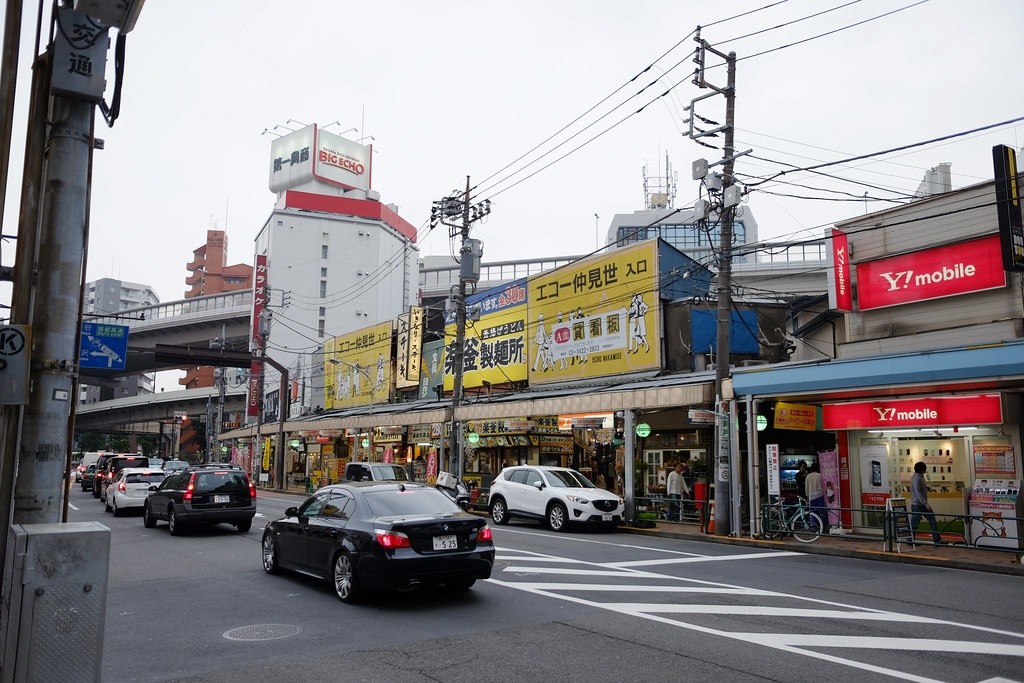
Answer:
[329,358,372,462]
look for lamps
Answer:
[934,430,943,438]
[999,426,1006,437]
[635,409,651,438]
[361,432,370,448]
[261,119,376,143]
[466,423,479,444]
[757,409,767,431]
[292,439,300,448]
[877,429,884,439]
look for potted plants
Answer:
[638,513,657,528]
[635,459,648,474]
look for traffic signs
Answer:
[79,323,130,370]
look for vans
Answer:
[92,454,120,498]
[81,452,113,474]
[342,462,412,483]
[99,456,149,502]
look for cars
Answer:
[261,481,496,604]
[163,461,191,475]
[148,459,164,468]
[488,464,625,532]
[74,464,82,482]
[81,463,96,491]
[105,468,167,517]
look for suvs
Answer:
[143,462,256,536]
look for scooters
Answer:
[435,474,476,512]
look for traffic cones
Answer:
[708,504,717,533]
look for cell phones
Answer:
[898,449,951,480]
[872,460,881,486]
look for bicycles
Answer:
[760,495,823,543]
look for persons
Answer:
[667,463,691,522]
[795,460,809,501]
[907,462,947,546]
[805,463,829,534]
[681,465,694,488]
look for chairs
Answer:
[205,474,232,489]
[127,474,137,482]
[148,473,160,481]
